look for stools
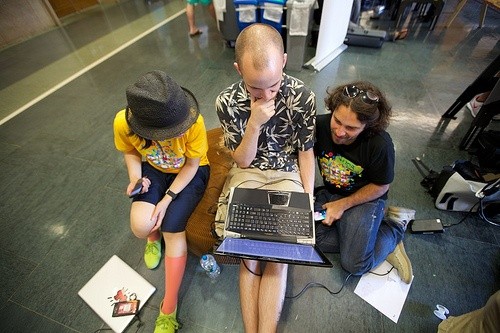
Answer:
[186,128,242,264]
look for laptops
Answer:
[214,187,334,268]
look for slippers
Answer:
[189,30,203,37]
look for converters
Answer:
[409,218,445,234]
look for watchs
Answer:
[166,189,177,200]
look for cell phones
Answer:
[112,300,140,317]
[314,208,327,221]
[129,175,148,198]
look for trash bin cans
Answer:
[213,1,285,48]
[285,0,316,72]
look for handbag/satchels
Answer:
[431,160,500,219]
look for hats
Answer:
[125,70,200,141]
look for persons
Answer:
[313,81,416,284]
[215,23,316,333]
[466,91,491,118]
[187,0,216,37]
[113,70,210,333]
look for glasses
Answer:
[340,84,381,112]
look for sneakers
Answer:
[144,229,162,269]
[385,240,412,285]
[154,299,178,333]
[387,206,416,231]
